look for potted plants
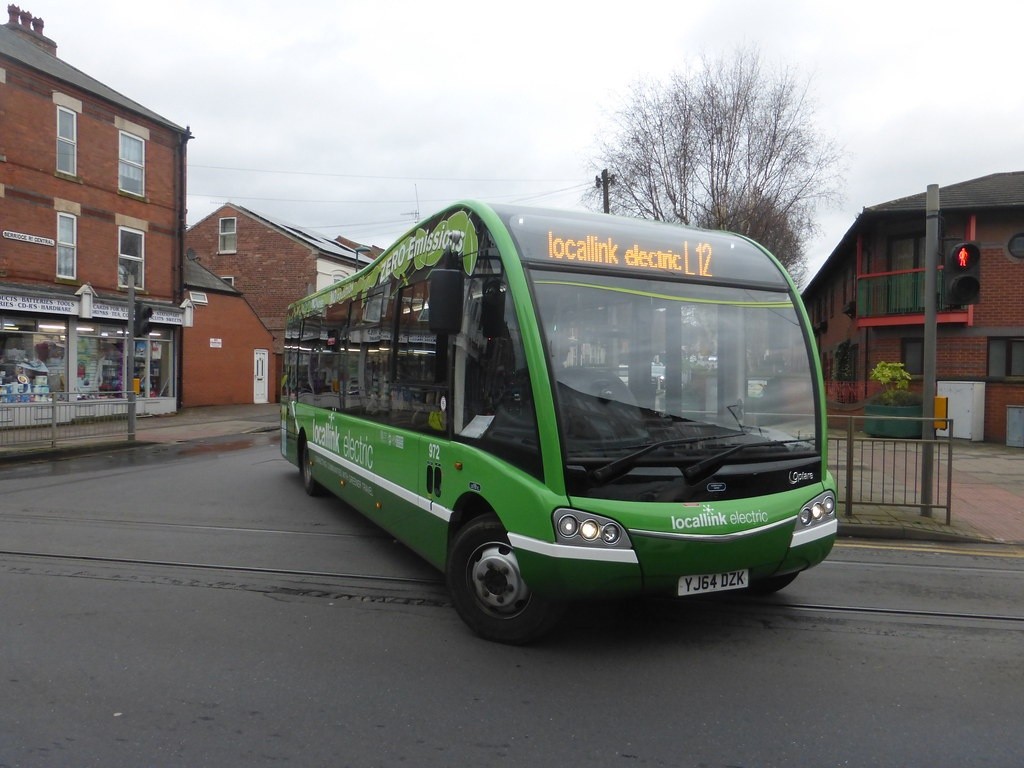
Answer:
[863,360,922,438]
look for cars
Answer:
[709,356,717,363]
[650,366,666,387]
[618,365,629,387]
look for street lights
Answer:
[354,247,371,273]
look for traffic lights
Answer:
[942,238,980,306]
[134,303,153,338]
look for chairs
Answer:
[568,370,650,450]
[362,402,442,428]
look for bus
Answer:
[278,199,839,645]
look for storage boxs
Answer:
[134,342,162,359]
